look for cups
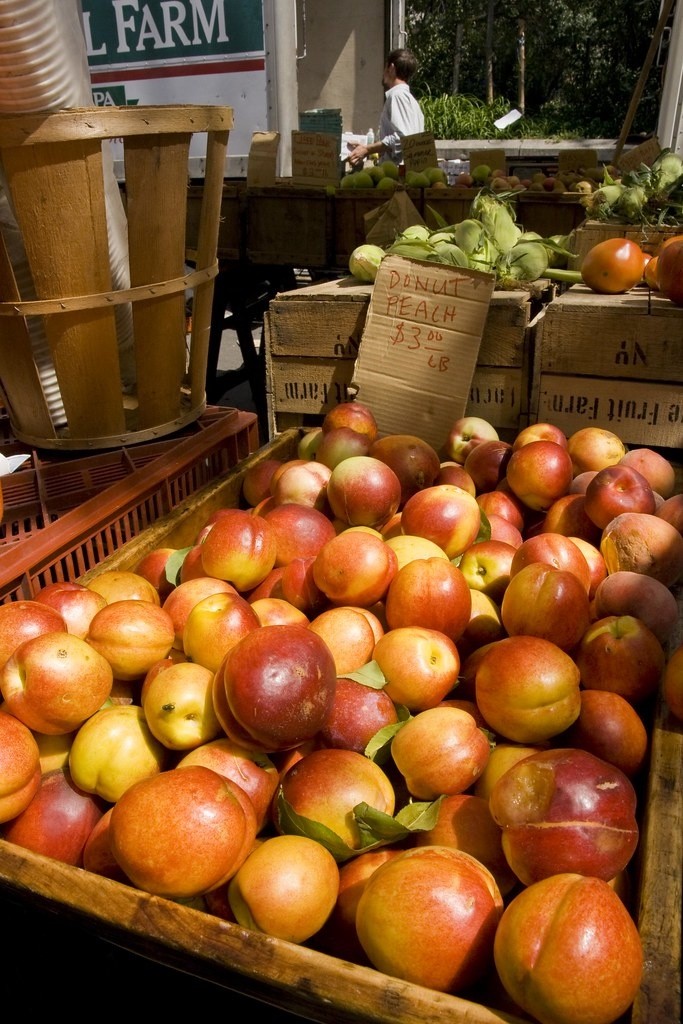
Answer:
[0,0,136,430]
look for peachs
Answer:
[0,402,682,1023]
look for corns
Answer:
[350,190,571,286]
[588,150,683,224]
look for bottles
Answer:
[367,124,381,145]
[437,155,470,186]
[494,107,523,131]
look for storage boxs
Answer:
[0,180,683,1024]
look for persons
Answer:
[347,48,424,175]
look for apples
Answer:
[339,161,620,194]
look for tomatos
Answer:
[581,234,683,305]
